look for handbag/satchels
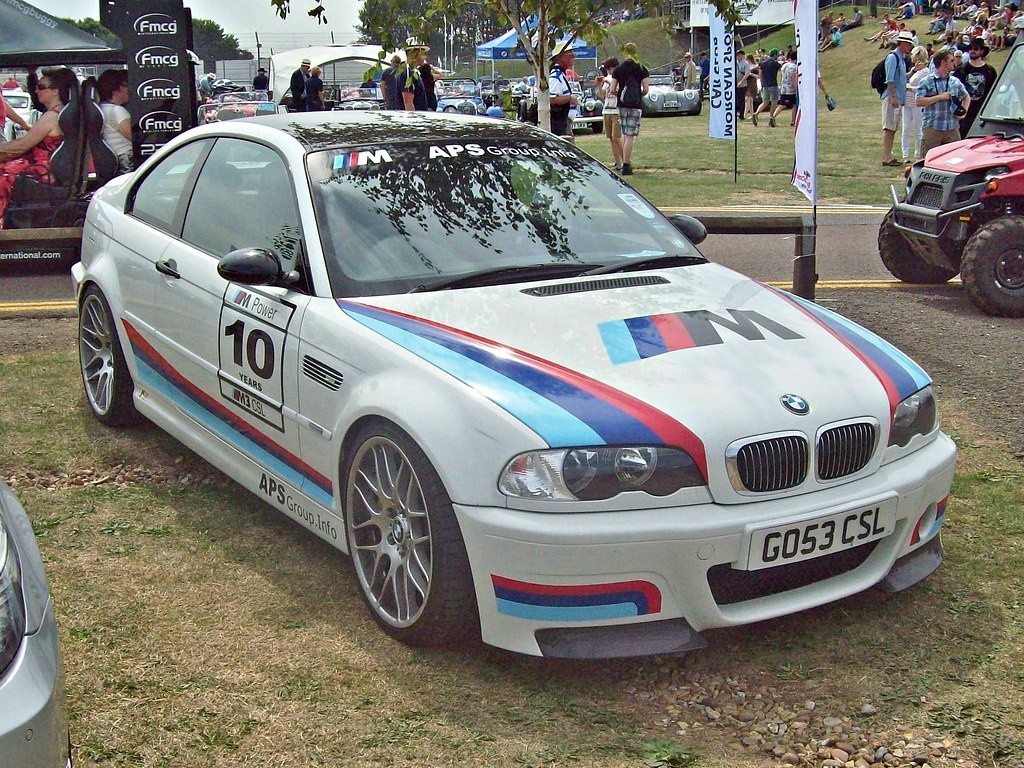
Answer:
[752,97,763,106]
[826,96,837,111]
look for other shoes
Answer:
[770,117,776,127]
[753,113,758,126]
[864,37,897,50]
[616,163,623,169]
[621,163,632,175]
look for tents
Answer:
[476,13,598,107]
[266,44,410,107]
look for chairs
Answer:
[6,78,84,228]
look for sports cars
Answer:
[638,75,702,116]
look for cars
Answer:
[2,90,43,125]
[196,84,288,124]
[433,77,502,118]
[877,16,1023,320]
[330,88,388,110]
[516,81,604,135]
[71,110,955,659]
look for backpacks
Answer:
[871,52,899,96]
[620,62,642,106]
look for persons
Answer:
[611,43,649,175]
[396,36,428,111]
[601,3,646,29]
[680,53,696,89]
[595,57,625,171]
[700,53,710,101]
[545,39,579,137]
[736,0,1024,166]
[518,76,528,94]
[291,59,311,112]
[381,55,401,110]
[201,73,215,99]
[98,69,134,174]
[305,66,326,112]
[0,65,87,231]
[418,64,443,112]
[359,77,377,98]
[253,68,268,94]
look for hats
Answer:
[893,32,914,44]
[769,48,780,55]
[301,59,311,66]
[967,38,990,57]
[899,23,905,27]
[549,41,578,61]
[737,50,745,55]
[257,68,267,73]
[403,36,430,52]
[207,72,216,80]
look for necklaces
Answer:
[52,103,63,110]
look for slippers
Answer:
[882,157,903,166]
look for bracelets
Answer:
[570,94,574,103]
[892,95,896,98]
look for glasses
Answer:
[969,47,980,51]
[209,77,213,80]
[944,59,955,62]
[954,56,961,58]
[684,57,690,58]
[35,83,58,90]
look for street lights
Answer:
[257,44,262,71]
